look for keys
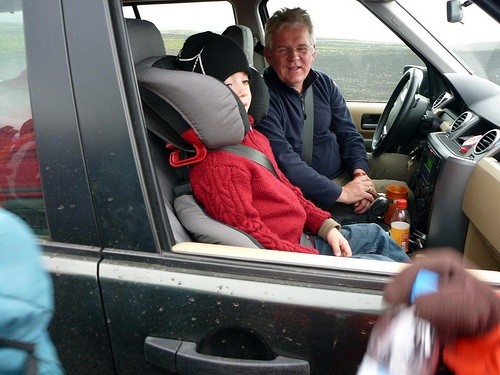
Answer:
[407,146,418,171]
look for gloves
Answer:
[381,257,500,344]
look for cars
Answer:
[0,0,500,375]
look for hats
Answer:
[175,31,252,87]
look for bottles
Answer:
[390,199,411,253]
[354,267,441,375]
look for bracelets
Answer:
[353,172,367,178]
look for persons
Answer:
[357,248,500,375]
[176,31,415,265]
[255,9,378,214]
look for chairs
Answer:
[125,19,177,216]
[221,25,254,68]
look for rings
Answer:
[366,186,372,192]
